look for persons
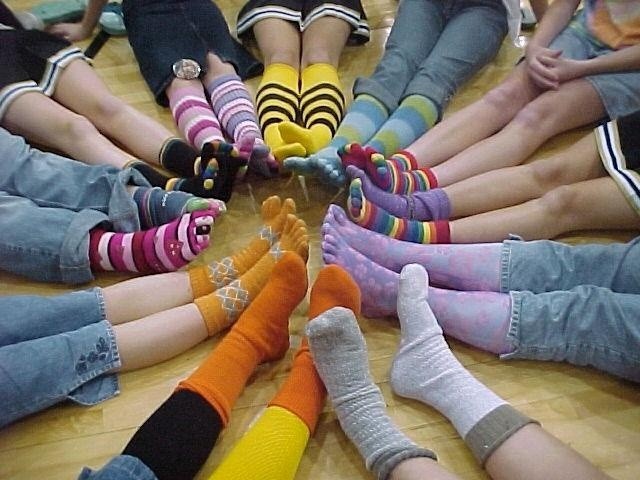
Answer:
[1,0,640,480]
